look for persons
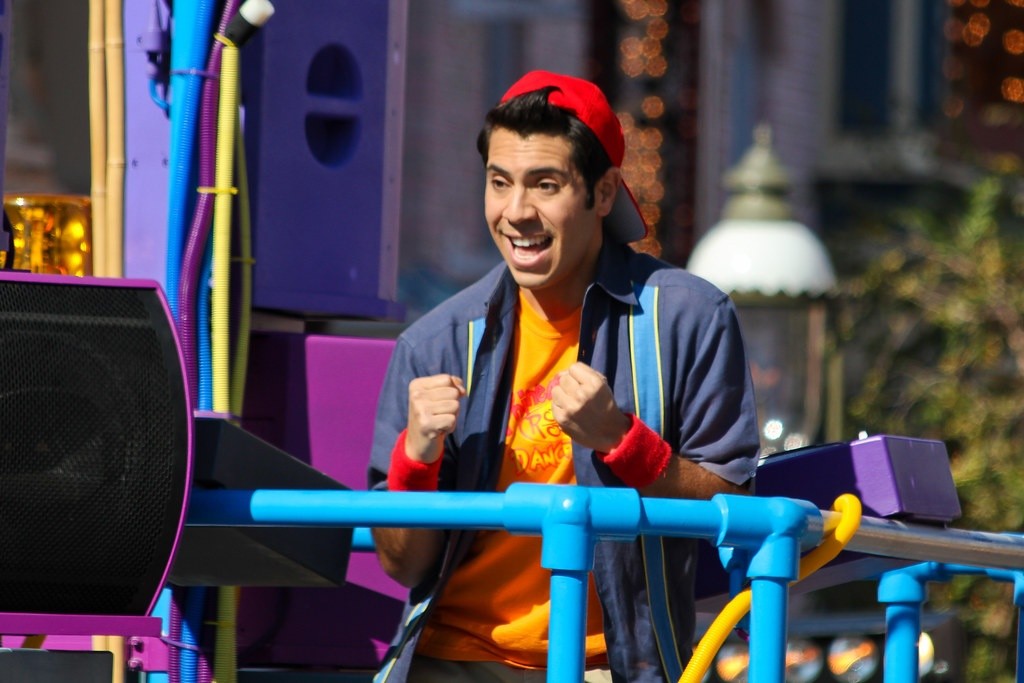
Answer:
[364,69,760,683]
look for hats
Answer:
[500,69,652,242]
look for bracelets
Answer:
[385,428,444,493]
[597,411,673,490]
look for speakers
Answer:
[239,0,407,302]
[0,272,195,616]
